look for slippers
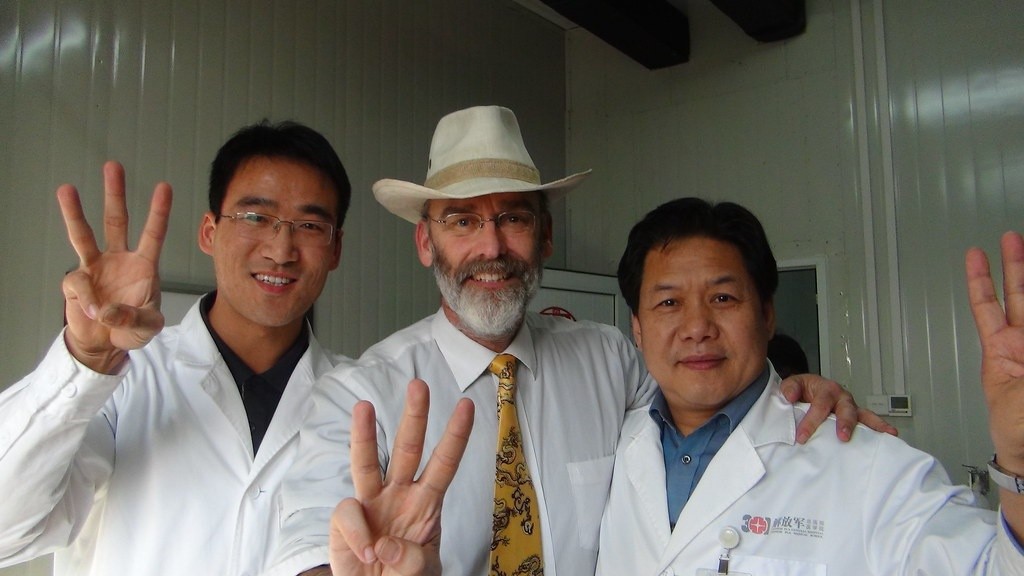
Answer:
[424,210,544,233]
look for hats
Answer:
[371,106,593,225]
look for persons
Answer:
[593,197,1024,576]
[0,120,353,576]
[767,335,809,379]
[260,105,898,576]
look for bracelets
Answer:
[987,453,1024,494]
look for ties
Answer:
[488,353,543,576]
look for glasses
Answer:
[218,212,343,248]
